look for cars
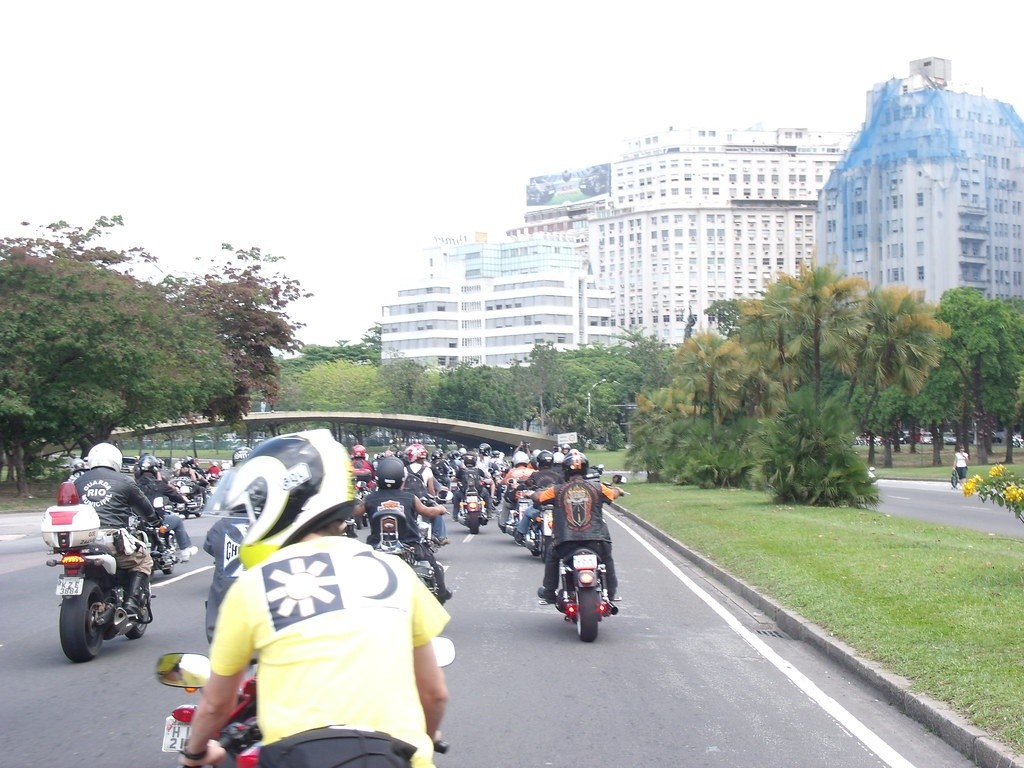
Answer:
[969,431,1001,444]
[121,457,138,473]
[898,431,912,444]
[943,433,956,444]
[921,432,933,444]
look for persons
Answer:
[525,451,624,603]
[71,443,164,623]
[345,439,578,547]
[203,487,263,665]
[953,446,969,486]
[133,453,234,509]
[67,458,89,483]
[128,457,198,563]
[183,431,451,768]
[352,456,452,601]
[613,474,622,484]
[156,652,207,688]
[233,446,253,467]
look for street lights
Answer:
[588,379,606,416]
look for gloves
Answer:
[156,517,164,528]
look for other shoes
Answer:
[434,537,450,546]
[497,519,507,534]
[514,531,522,546]
[346,515,368,538]
[439,587,453,606]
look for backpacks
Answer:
[405,464,427,492]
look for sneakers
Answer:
[537,586,556,603]
[180,546,199,561]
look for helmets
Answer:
[530,443,580,464]
[375,457,405,490]
[221,428,355,571]
[403,443,429,464]
[431,448,467,461]
[562,452,588,482]
[491,450,505,461]
[139,452,164,474]
[88,443,123,473]
[536,451,554,470]
[365,450,403,461]
[479,443,491,456]
[511,451,531,467]
[232,446,253,467]
[70,456,90,472]
[353,445,365,458]
[464,452,477,467]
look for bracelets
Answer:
[179,739,207,761]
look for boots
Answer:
[123,571,148,623]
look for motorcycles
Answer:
[41,482,165,664]
[345,469,371,536]
[527,475,631,641]
[157,637,456,768]
[143,486,189,578]
[483,470,555,561]
[453,468,495,535]
[366,491,453,600]
[169,474,219,518]
[435,470,459,504]
[415,497,432,547]
